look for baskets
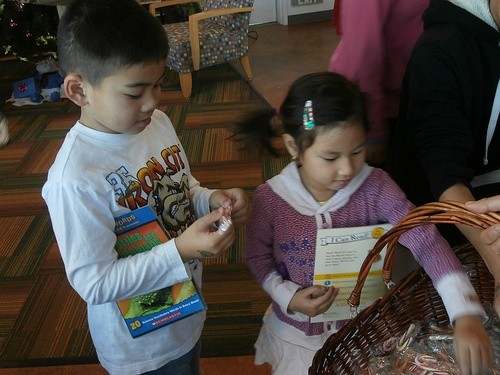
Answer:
[307,200,499,374]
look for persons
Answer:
[327,0,500,323]
[38,0,253,375]
[239,71,493,375]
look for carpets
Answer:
[0,61,293,368]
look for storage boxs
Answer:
[11,76,40,98]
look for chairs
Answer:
[149,0,255,99]
